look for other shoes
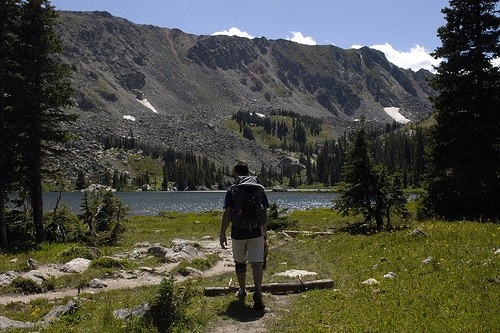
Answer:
[252,295,262,303]
[236,291,246,303]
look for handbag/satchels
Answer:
[263,241,270,270]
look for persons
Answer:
[219,160,269,304]
